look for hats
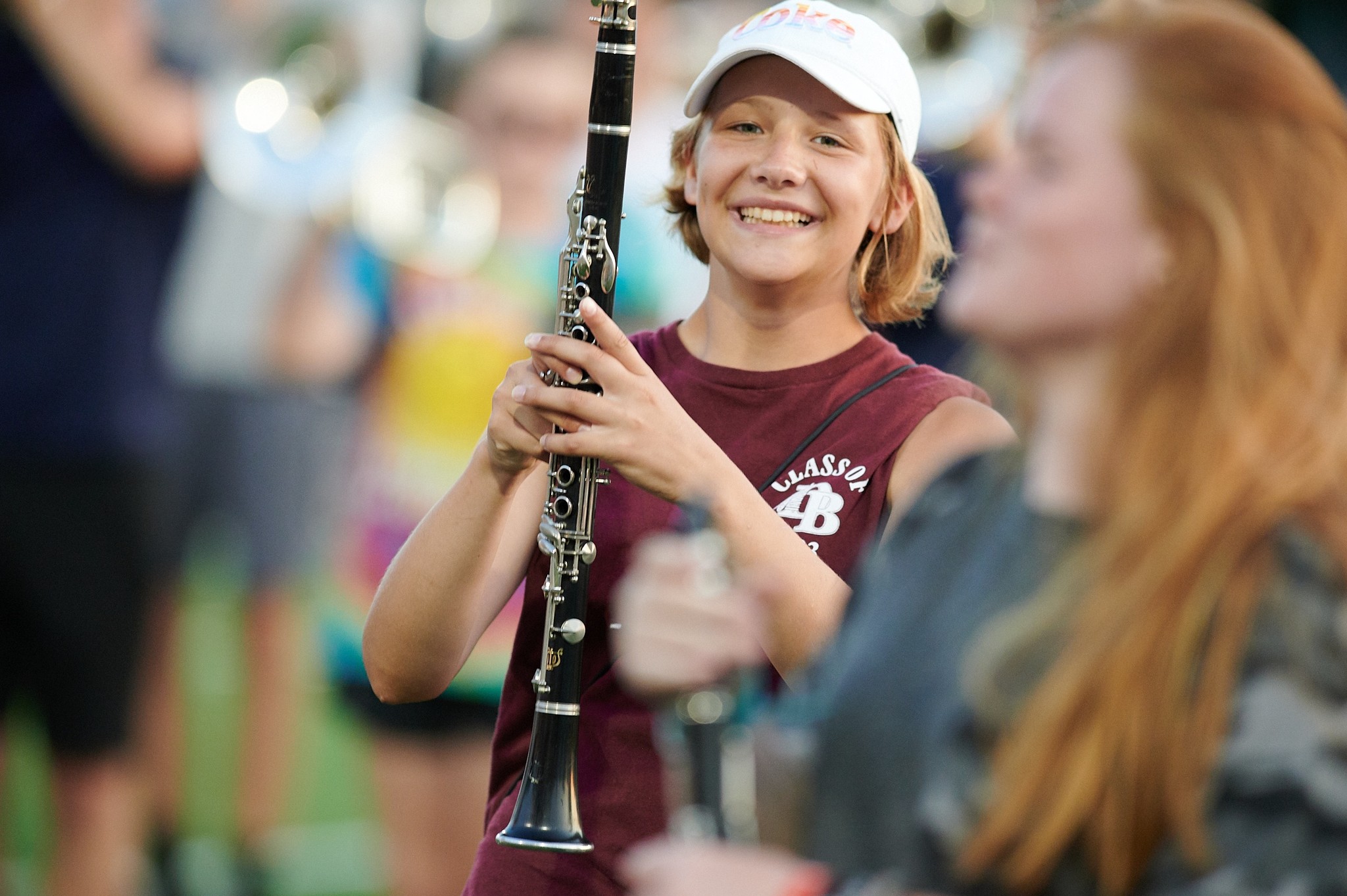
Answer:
[684,0,920,161]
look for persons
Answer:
[0,0,740,896]
[615,0,1345,896]
[866,4,999,378]
[361,0,1017,896]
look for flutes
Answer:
[490,0,641,858]
